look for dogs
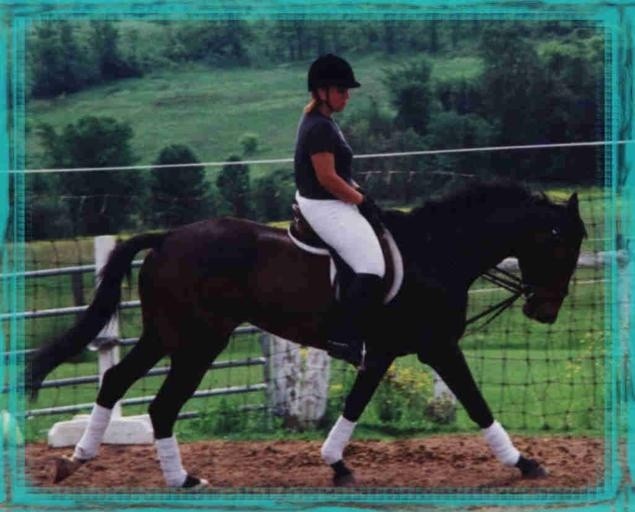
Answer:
[23,175,588,491]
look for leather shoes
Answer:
[356,197,381,223]
[355,187,375,204]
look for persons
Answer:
[291,54,389,362]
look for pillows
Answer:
[306,54,362,93]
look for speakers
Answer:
[326,271,383,352]
